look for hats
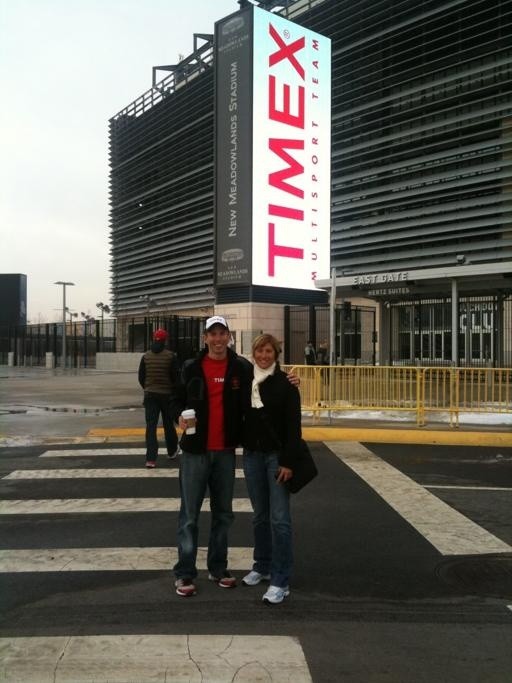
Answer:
[154,330,168,341]
[204,316,229,331]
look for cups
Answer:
[181,408,198,435]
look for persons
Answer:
[135,327,178,469]
[173,315,302,597]
[304,341,330,380]
[236,333,301,605]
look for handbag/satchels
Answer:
[274,439,317,494]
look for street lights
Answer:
[64,305,78,369]
[140,294,158,352]
[81,309,94,369]
[96,300,112,351]
[52,279,77,371]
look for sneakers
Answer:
[145,460,156,466]
[167,445,181,458]
[262,586,290,604]
[208,574,236,588]
[174,578,196,596]
[242,570,271,586]
